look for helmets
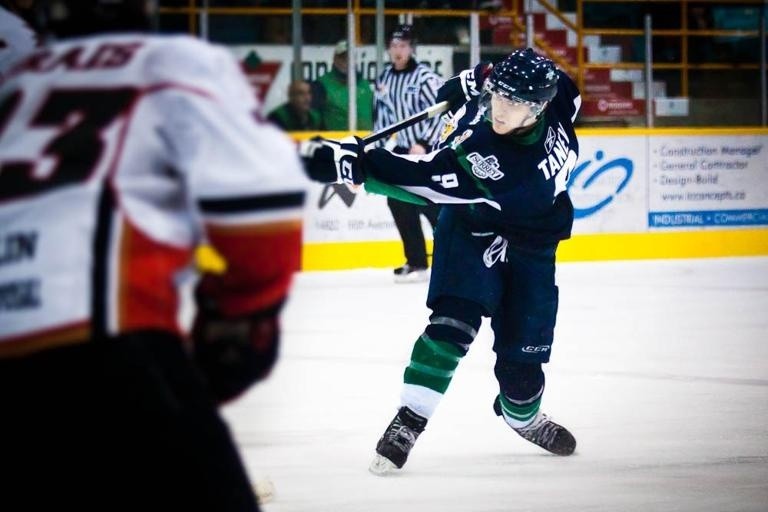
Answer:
[483,47,559,105]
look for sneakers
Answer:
[394,264,428,275]
[376,407,427,471]
[515,413,576,454]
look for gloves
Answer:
[299,136,364,186]
[435,63,493,120]
[190,310,279,407]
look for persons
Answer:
[312,38,373,130]
[372,23,447,282]
[267,79,323,131]
[0,33,309,511]
[295,46,582,474]
